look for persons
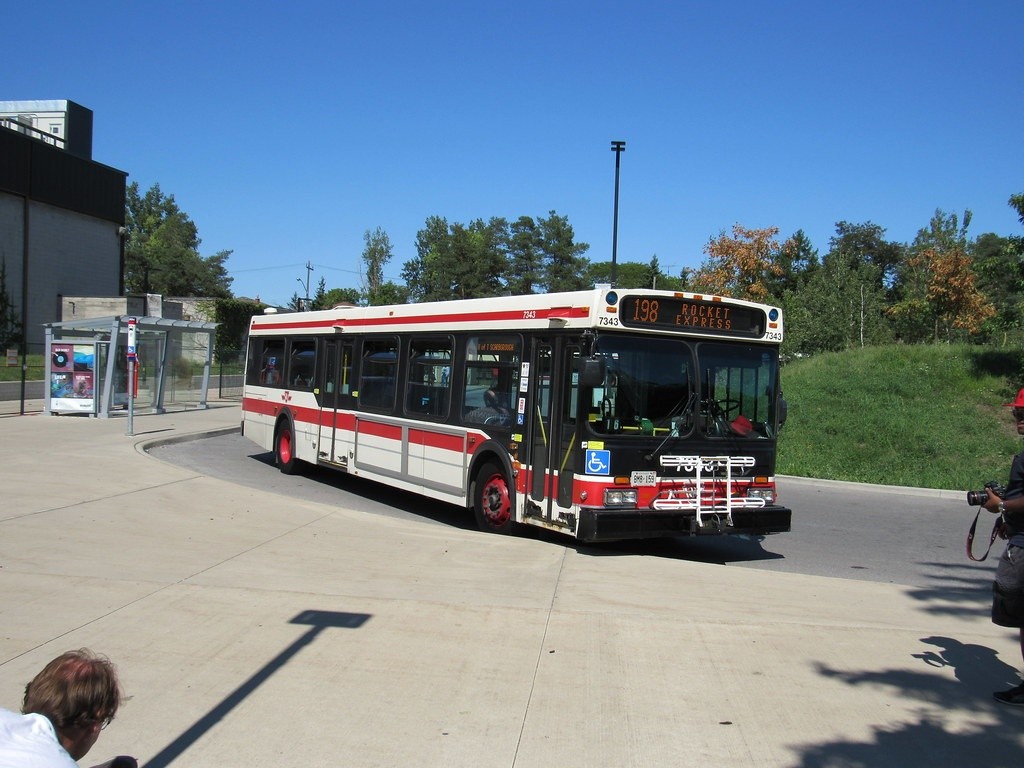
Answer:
[259,357,281,384]
[429,365,450,387]
[982,387,1024,706]
[466,387,510,427]
[1,647,124,768]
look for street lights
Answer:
[610,140,626,288]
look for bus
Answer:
[238,281,793,544]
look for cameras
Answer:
[967,481,1006,506]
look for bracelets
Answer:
[999,500,1005,513]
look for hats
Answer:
[731,415,753,435]
[1002,389,1024,407]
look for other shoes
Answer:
[994,681,1024,706]
